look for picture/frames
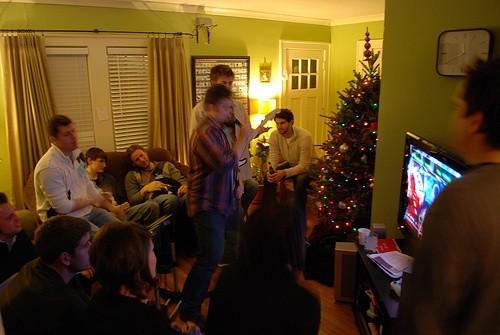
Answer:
[190,55,251,120]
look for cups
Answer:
[357,228,371,245]
[364,234,379,253]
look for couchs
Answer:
[23,146,188,238]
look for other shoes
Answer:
[179,305,207,326]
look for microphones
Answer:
[232,117,244,128]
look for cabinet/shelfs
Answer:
[351,238,415,335]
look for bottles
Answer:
[269,162,275,174]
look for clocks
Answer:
[436,27,491,77]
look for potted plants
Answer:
[252,143,270,203]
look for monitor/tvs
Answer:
[397,131,476,255]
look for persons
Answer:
[82,146,161,226]
[86,220,201,335]
[399,56,500,335]
[125,143,194,258]
[0,214,93,335]
[32,113,131,246]
[263,109,318,230]
[0,192,37,284]
[179,83,253,330]
[15,209,40,239]
[188,64,259,242]
[203,201,321,335]
